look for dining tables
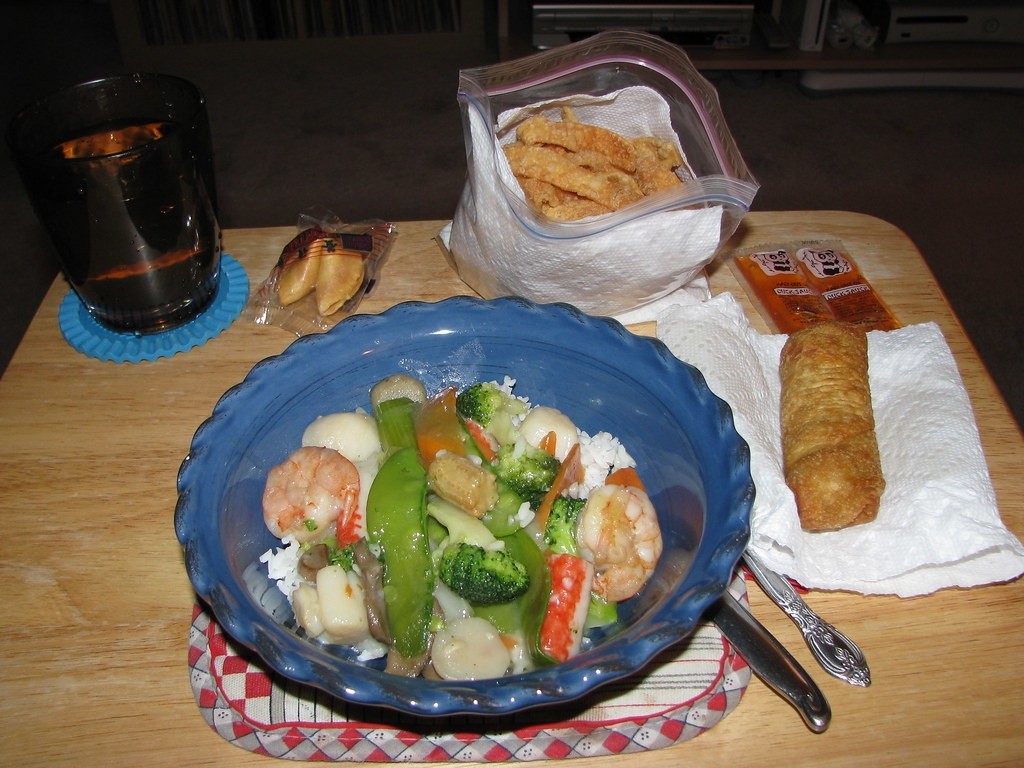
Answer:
[0,212,1024,768]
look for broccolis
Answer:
[492,434,559,496]
[453,378,526,421]
[430,496,529,605]
[537,497,593,552]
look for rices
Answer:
[259,376,664,680]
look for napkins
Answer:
[440,85,711,326]
[658,291,1024,598]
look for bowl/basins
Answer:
[172,296,755,719]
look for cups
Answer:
[15,71,223,335]
